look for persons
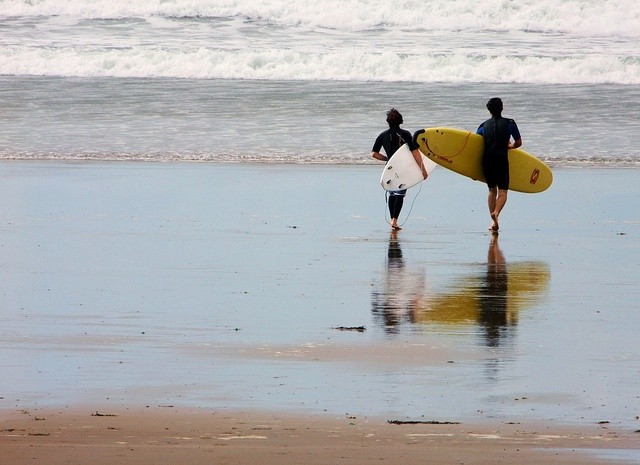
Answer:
[370,107,428,231]
[475,98,522,231]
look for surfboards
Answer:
[380,143,437,191]
[412,128,553,193]
